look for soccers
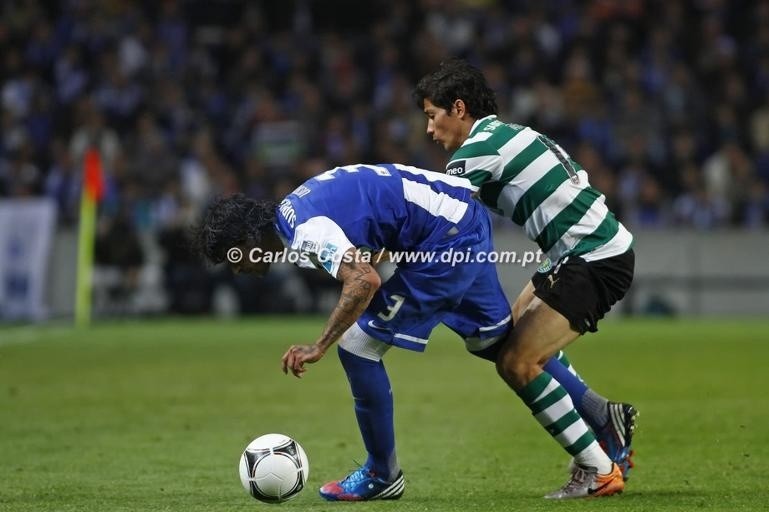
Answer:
[239,433,308,504]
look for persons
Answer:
[414,62,637,504]
[191,161,640,504]
[1,1,768,318]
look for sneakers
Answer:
[597,436,634,481]
[318,460,405,501]
[594,400,639,466]
[543,459,624,499]
